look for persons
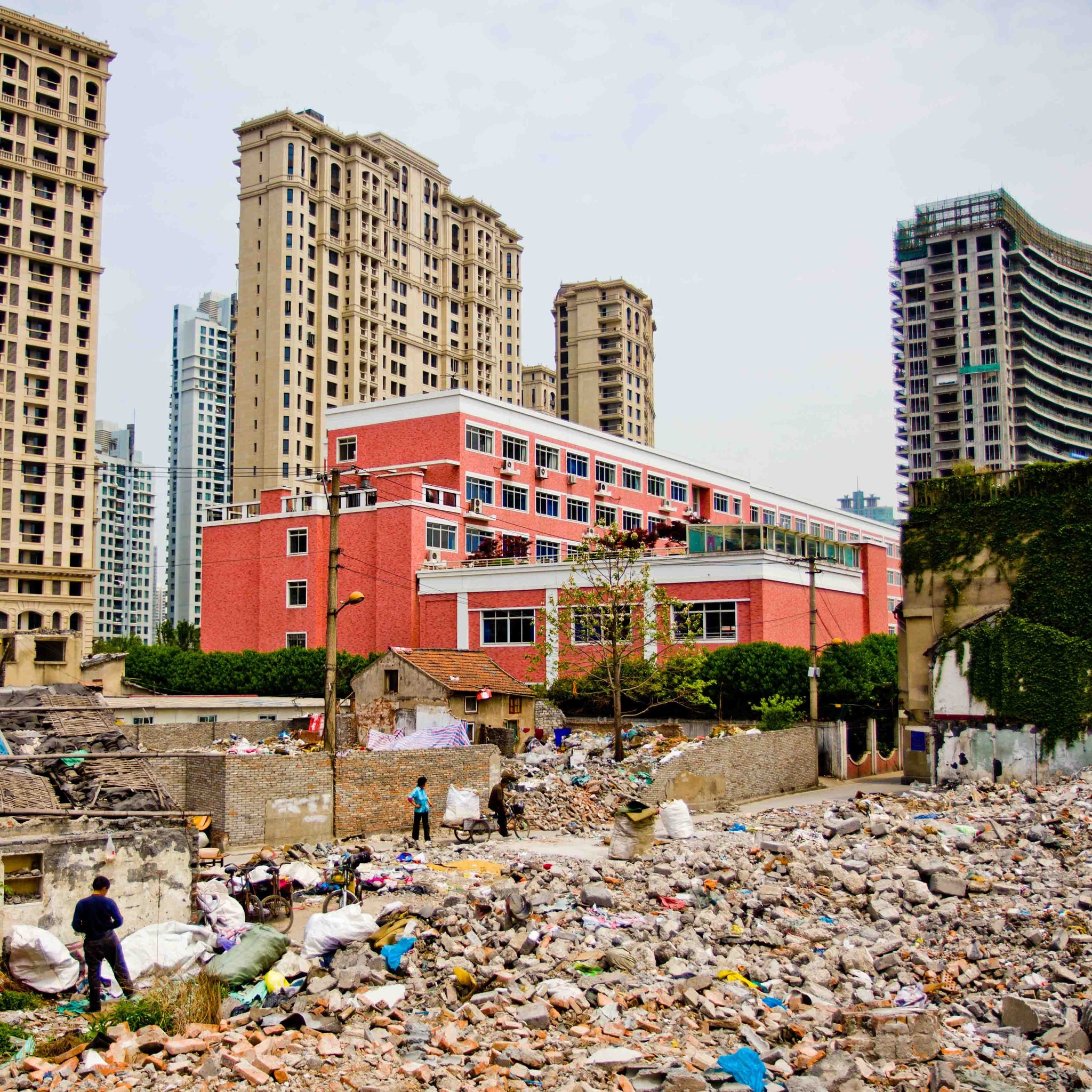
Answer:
[488,777,513,837]
[71,876,134,1013]
[407,777,434,844]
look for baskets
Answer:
[510,804,524,814]
[324,868,352,886]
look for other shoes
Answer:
[83,1008,101,1013]
[503,833,513,837]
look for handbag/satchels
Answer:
[105,834,117,863]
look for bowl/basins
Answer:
[199,847,219,858]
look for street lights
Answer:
[322,591,365,753]
[809,637,844,788]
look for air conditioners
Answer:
[586,528,595,533]
[536,466,547,477]
[427,550,440,562]
[739,520,746,524]
[598,482,607,490]
[503,460,514,470]
[685,505,692,512]
[470,498,482,513]
[662,498,669,507]
[569,475,576,482]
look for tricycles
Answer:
[223,857,294,933]
[440,795,529,844]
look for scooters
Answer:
[321,851,372,913]
